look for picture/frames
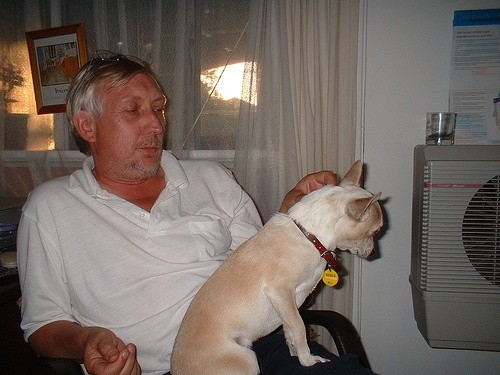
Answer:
[25,22,89,114]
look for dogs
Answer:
[170,160,383,375]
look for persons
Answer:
[17,53,372,375]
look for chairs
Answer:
[30,309,373,375]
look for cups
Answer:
[426,112,456,145]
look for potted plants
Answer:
[0,64,29,150]
